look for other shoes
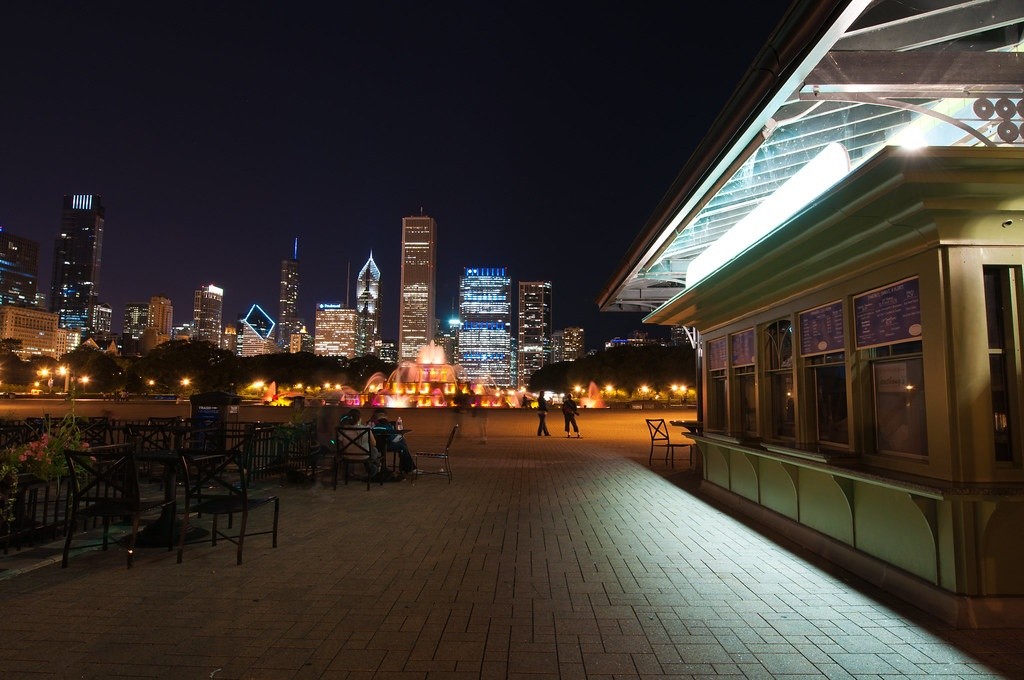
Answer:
[411,469,424,475]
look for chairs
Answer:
[646,417,694,472]
[0,414,415,567]
[415,424,459,485]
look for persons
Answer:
[472,374,498,446]
[522,394,528,410]
[562,393,581,438]
[368,407,423,476]
[338,407,387,478]
[534,390,551,437]
[115,388,130,403]
